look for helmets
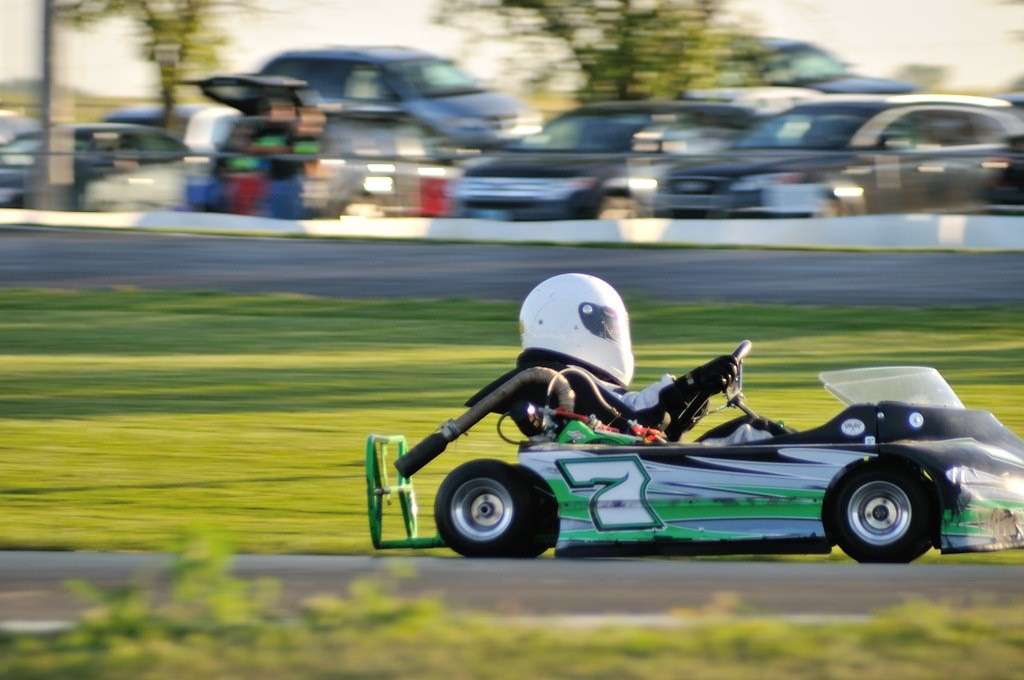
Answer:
[516,271,635,388]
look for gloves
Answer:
[676,354,738,404]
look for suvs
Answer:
[654,91,1023,220]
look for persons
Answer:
[516,274,743,442]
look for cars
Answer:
[1,48,827,228]
